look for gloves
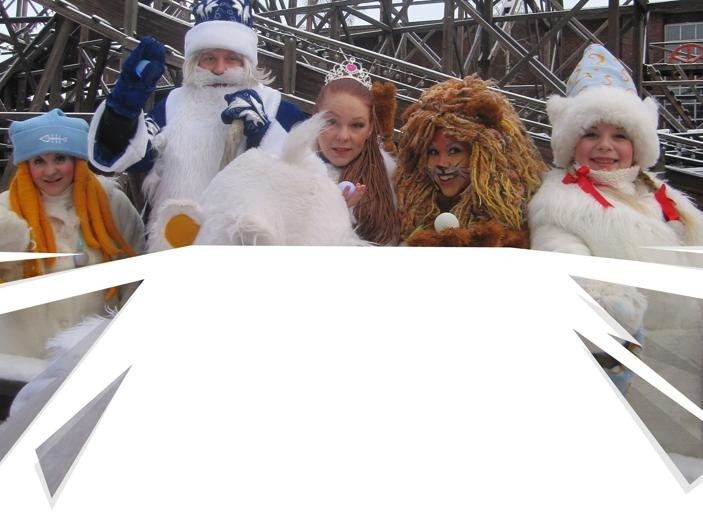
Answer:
[108,36,166,117]
[222,89,267,147]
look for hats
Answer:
[548,44,660,169]
[184,0,258,65]
[9,109,89,163]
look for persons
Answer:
[528,42,703,369]
[314,57,398,245]
[371,75,548,248]
[88,1,303,249]
[1,110,146,419]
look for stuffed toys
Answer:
[152,109,358,250]
